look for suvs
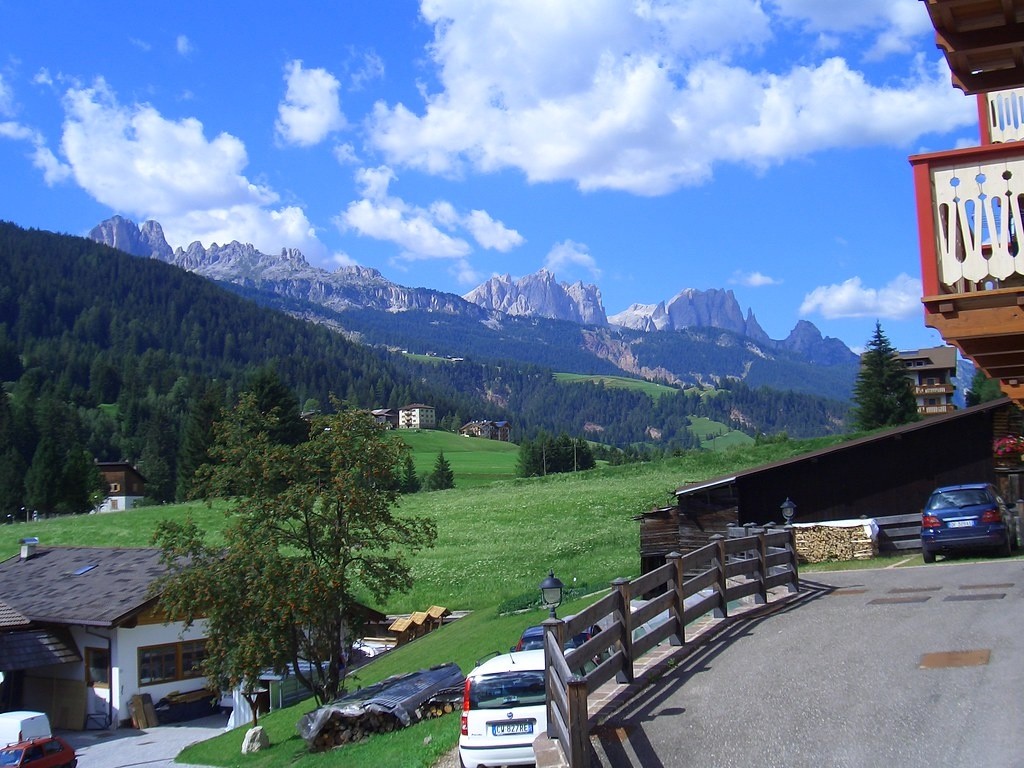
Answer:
[0,730,78,768]
[457,647,599,768]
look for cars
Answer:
[921,483,1017,563]
[510,614,604,680]
